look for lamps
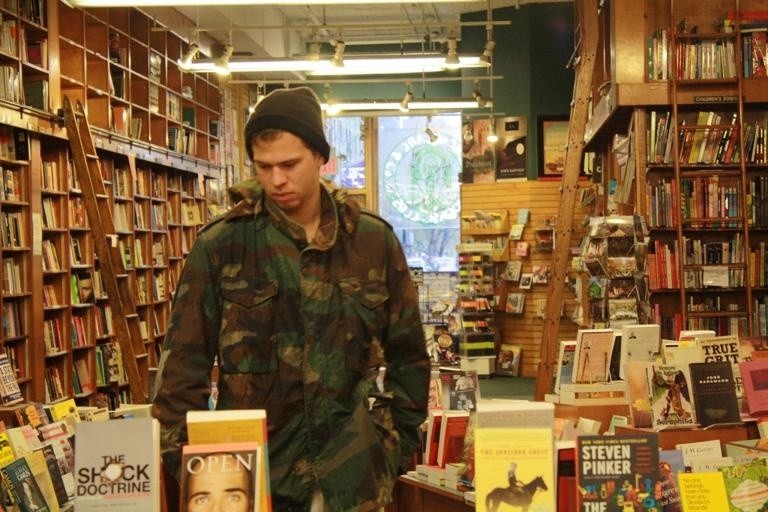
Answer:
[150,0,499,143]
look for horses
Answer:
[485,475,548,512]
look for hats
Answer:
[243,87,330,164]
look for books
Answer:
[409,208,549,376]
[641,19,767,512]
[0,0,273,512]
[401,324,662,512]
[565,133,645,333]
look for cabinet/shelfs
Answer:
[464,210,513,262]
[567,0,767,341]
[1,1,226,430]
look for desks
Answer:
[396,472,477,512]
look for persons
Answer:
[152,86,431,511]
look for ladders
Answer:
[670,0,754,337]
[63,95,149,404]
[535,0,600,401]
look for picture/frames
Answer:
[537,113,591,178]
